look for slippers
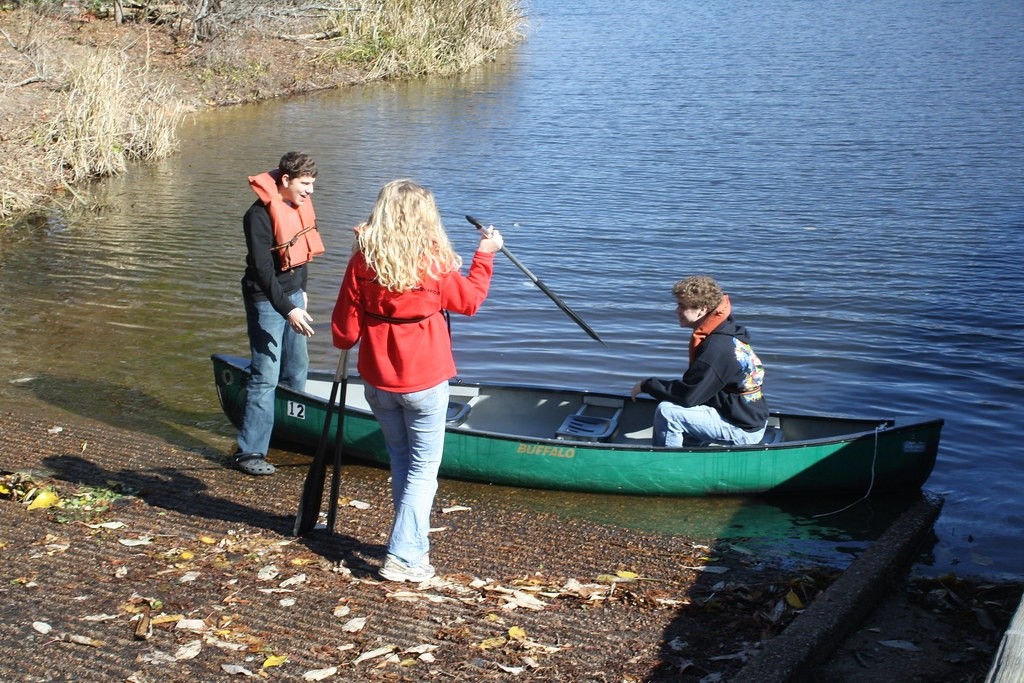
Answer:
[231,453,276,475]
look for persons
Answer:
[233,151,325,474]
[331,178,504,583]
[631,275,769,447]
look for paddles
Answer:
[465,213,609,347]
[291,344,353,536]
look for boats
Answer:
[211,352,946,498]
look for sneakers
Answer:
[378,553,435,582]
